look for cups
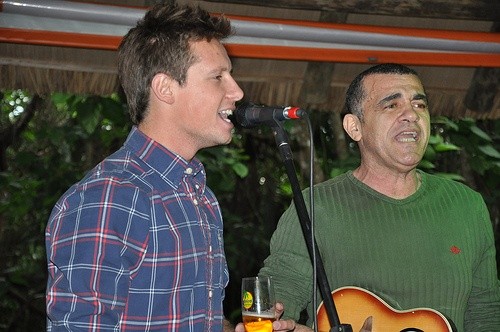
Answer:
[241,276,274,332]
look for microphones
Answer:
[235,103,304,127]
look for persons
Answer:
[46,1,294,332]
[258,63,500,331]
